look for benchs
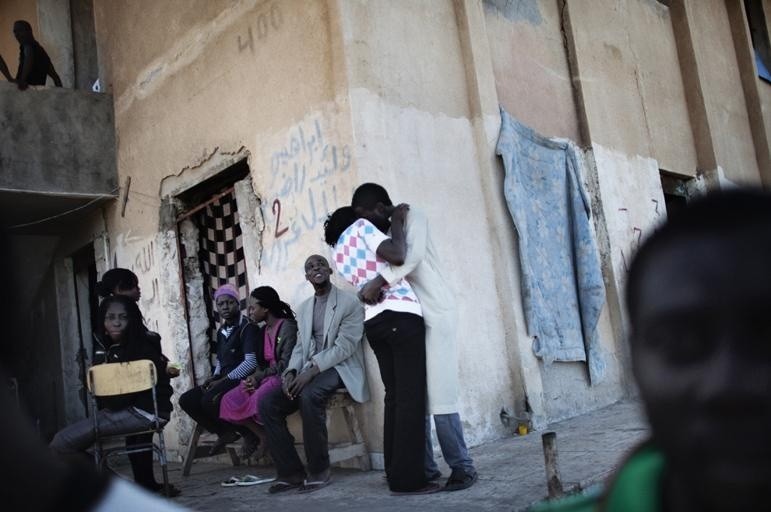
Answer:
[181,385,372,476]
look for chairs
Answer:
[86,359,171,500]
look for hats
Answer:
[214,284,240,304]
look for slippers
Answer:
[222,473,332,494]
[240,436,259,456]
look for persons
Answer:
[11,19,64,91]
[50,294,176,479]
[256,253,370,495]
[0,223,207,512]
[522,168,770,511]
[219,284,299,464]
[350,180,479,493]
[176,284,262,457]
[0,54,28,86]
[323,201,444,494]
[94,267,182,499]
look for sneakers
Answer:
[390,468,477,494]
[209,433,238,453]
